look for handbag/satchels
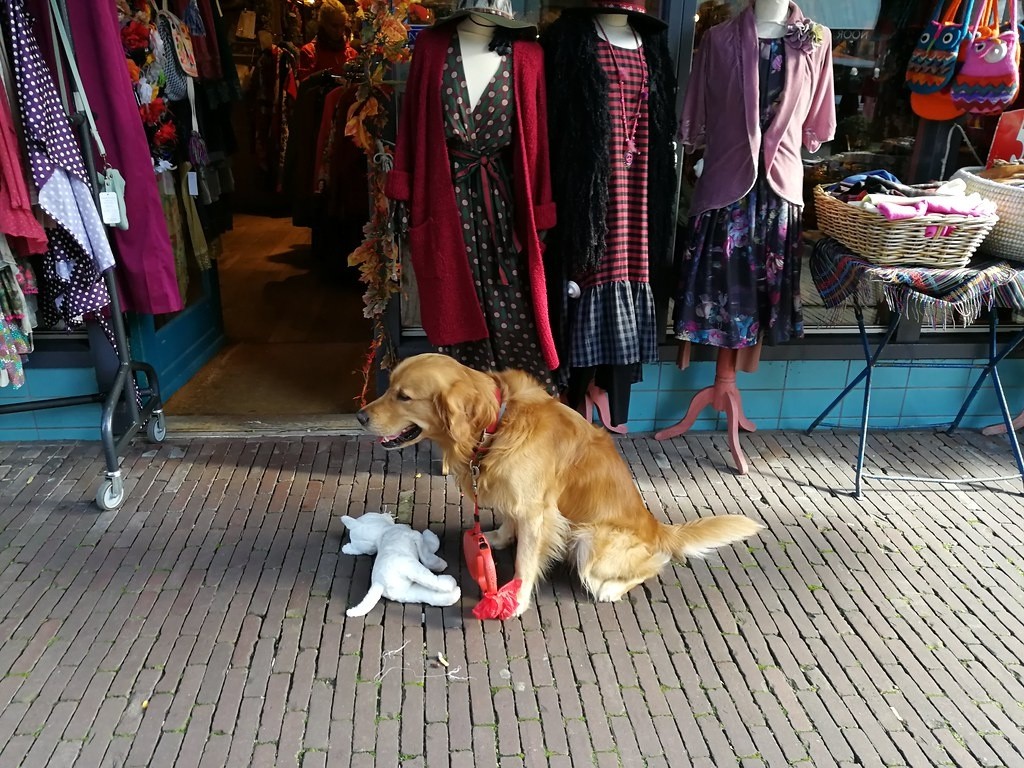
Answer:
[905,0,1024,120]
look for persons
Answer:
[405,0,668,374]
[687,0,830,357]
[296,0,369,88]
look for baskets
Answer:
[949,165,1024,264]
[813,183,999,268]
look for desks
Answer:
[800,231,1024,502]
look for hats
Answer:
[431,0,539,42]
[561,1,669,35]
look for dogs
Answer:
[356,352,766,621]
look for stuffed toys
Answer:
[340,511,461,618]
